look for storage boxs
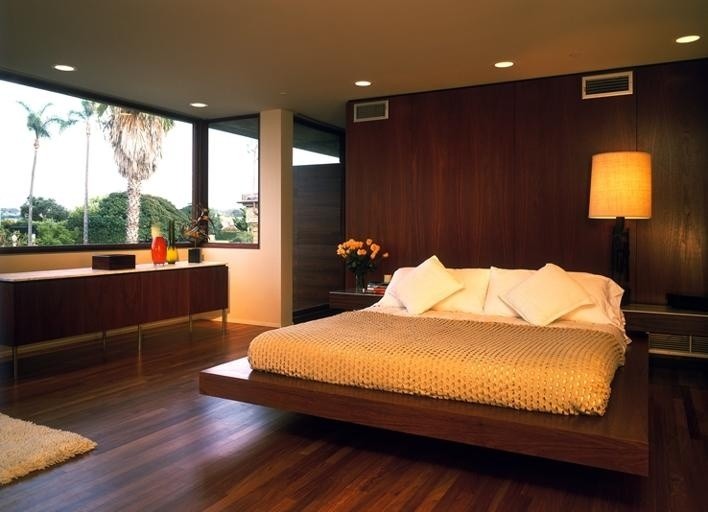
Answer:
[92,254,135,270]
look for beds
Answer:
[198,267,650,477]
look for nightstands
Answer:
[621,304,708,361]
[328,291,384,310]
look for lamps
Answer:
[589,151,653,306]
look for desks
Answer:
[0,261,227,376]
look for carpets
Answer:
[0,412,96,486]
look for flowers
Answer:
[337,239,389,276]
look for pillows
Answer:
[483,266,625,328]
[381,267,491,315]
[498,262,594,327]
[390,255,463,315]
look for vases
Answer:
[354,271,366,292]
[151,235,166,266]
[166,220,179,263]
